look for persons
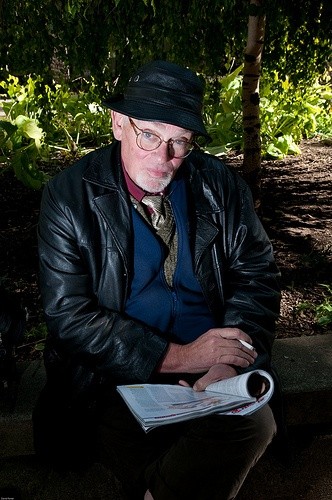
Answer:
[32,56,283,500]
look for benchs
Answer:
[0,332,332,500]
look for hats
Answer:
[104,61,213,145]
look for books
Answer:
[111,364,275,437]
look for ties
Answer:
[142,194,164,231]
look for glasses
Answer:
[126,117,196,159]
